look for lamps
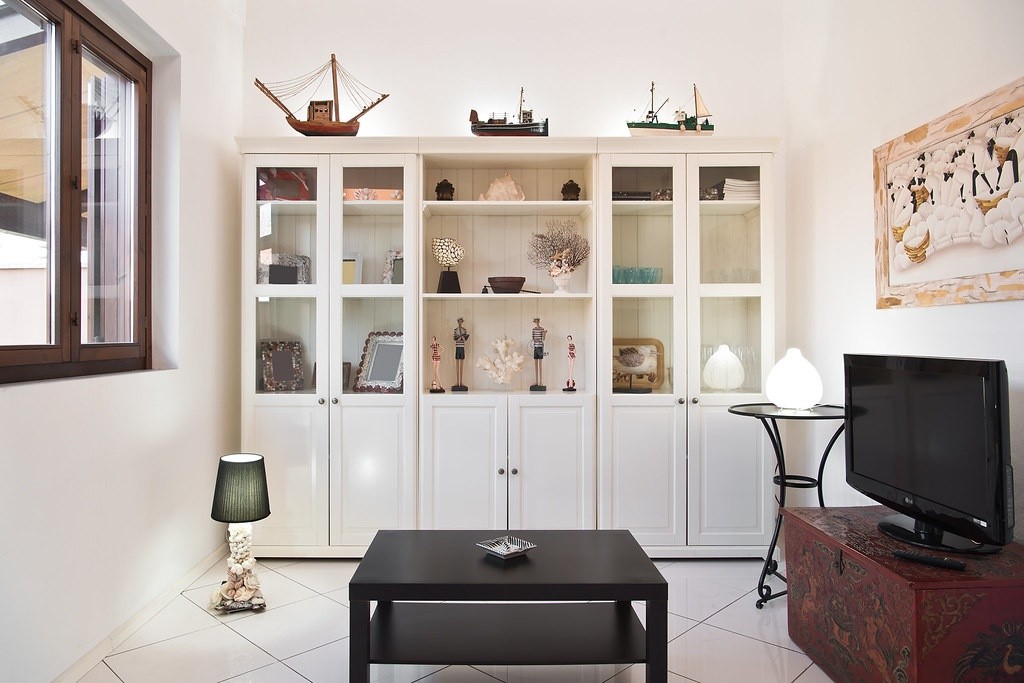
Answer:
[765,348,822,410]
[210,455,271,612]
[702,344,744,390]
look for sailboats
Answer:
[626,81,714,137]
[253,53,389,136]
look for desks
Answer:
[725,403,848,610]
[348,528,671,683]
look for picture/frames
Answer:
[353,331,405,392]
[383,248,401,284]
[343,252,362,284]
[257,252,310,284]
[257,168,309,201]
[873,75,1024,307]
[259,341,303,391]
[613,337,664,389]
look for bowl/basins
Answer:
[343,188,402,201]
[488,277,526,293]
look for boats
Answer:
[470,87,549,137]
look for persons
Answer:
[566,335,575,388]
[453,317,470,386]
[429,335,444,389]
[531,316,547,386]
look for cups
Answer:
[613,267,663,284]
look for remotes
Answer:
[891,548,967,571]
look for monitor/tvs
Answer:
[842,353,1015,556]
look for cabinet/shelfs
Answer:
[238,133,785,562]
[781,504,1023,683]
